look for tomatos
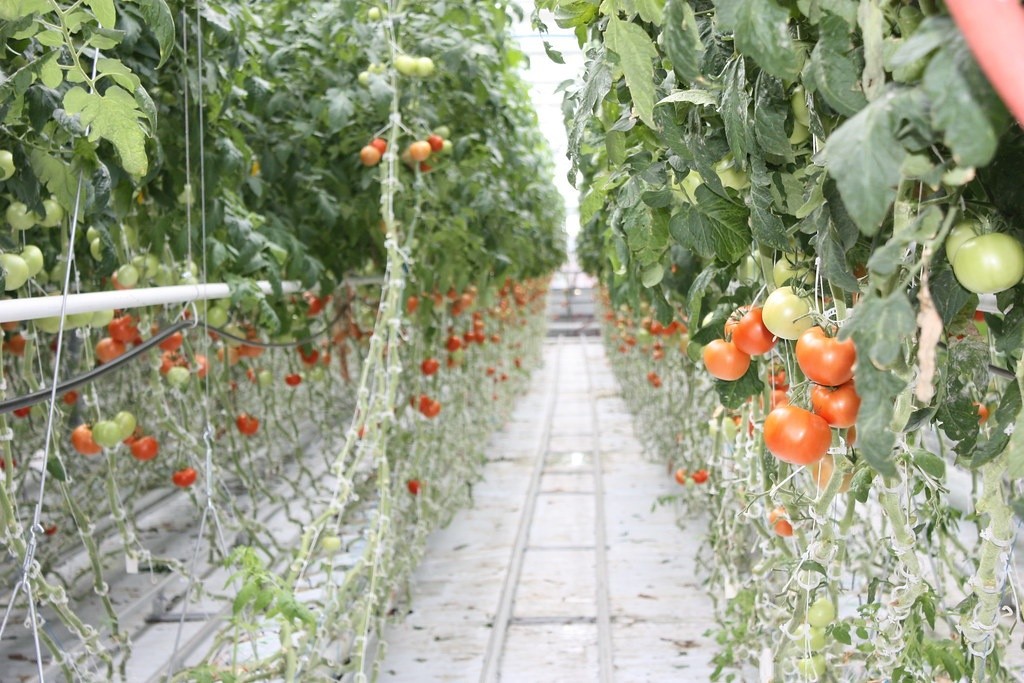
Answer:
[0,5,1022,536]
